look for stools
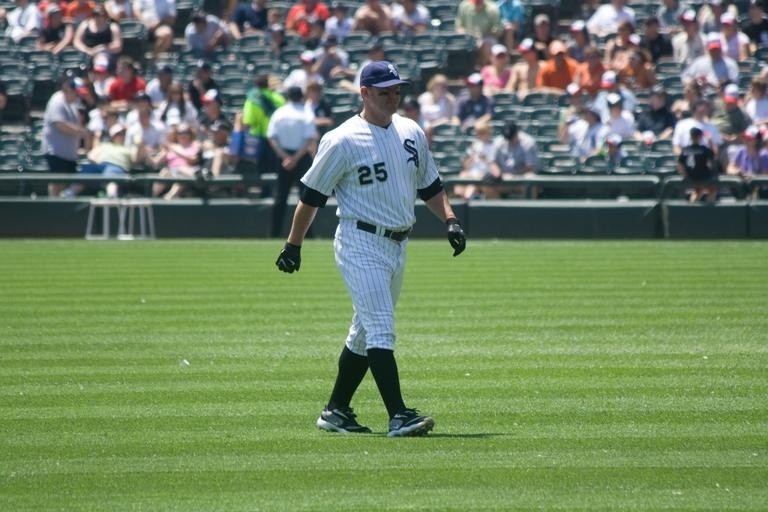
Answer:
[82,199,126,241]
[113,200,155,241]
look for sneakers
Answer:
[316,405,372,435]
[387,408,435,438]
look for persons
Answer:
[1,0,768,208]
[264,85,319,239]
[275,61,468,436]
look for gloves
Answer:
[275,241,301,274]
[446,218,466,256]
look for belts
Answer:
[357,220,412,241]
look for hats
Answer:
[683,9,765,143]
[55,51,322,140]
[466,13,566,87]
[360,60,411,89]
[566,15,673,144]
